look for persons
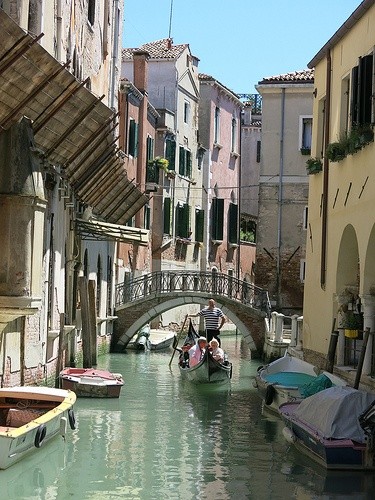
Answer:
[185,299,225,348]
[178,334,225,367]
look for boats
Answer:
[177,338,234,386]
[0,385,77,468]
[275,388,374,477]
[57,365,123,401]
[256,348,348,406]
[127,328,177,351]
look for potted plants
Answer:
[337,287,364,338]
